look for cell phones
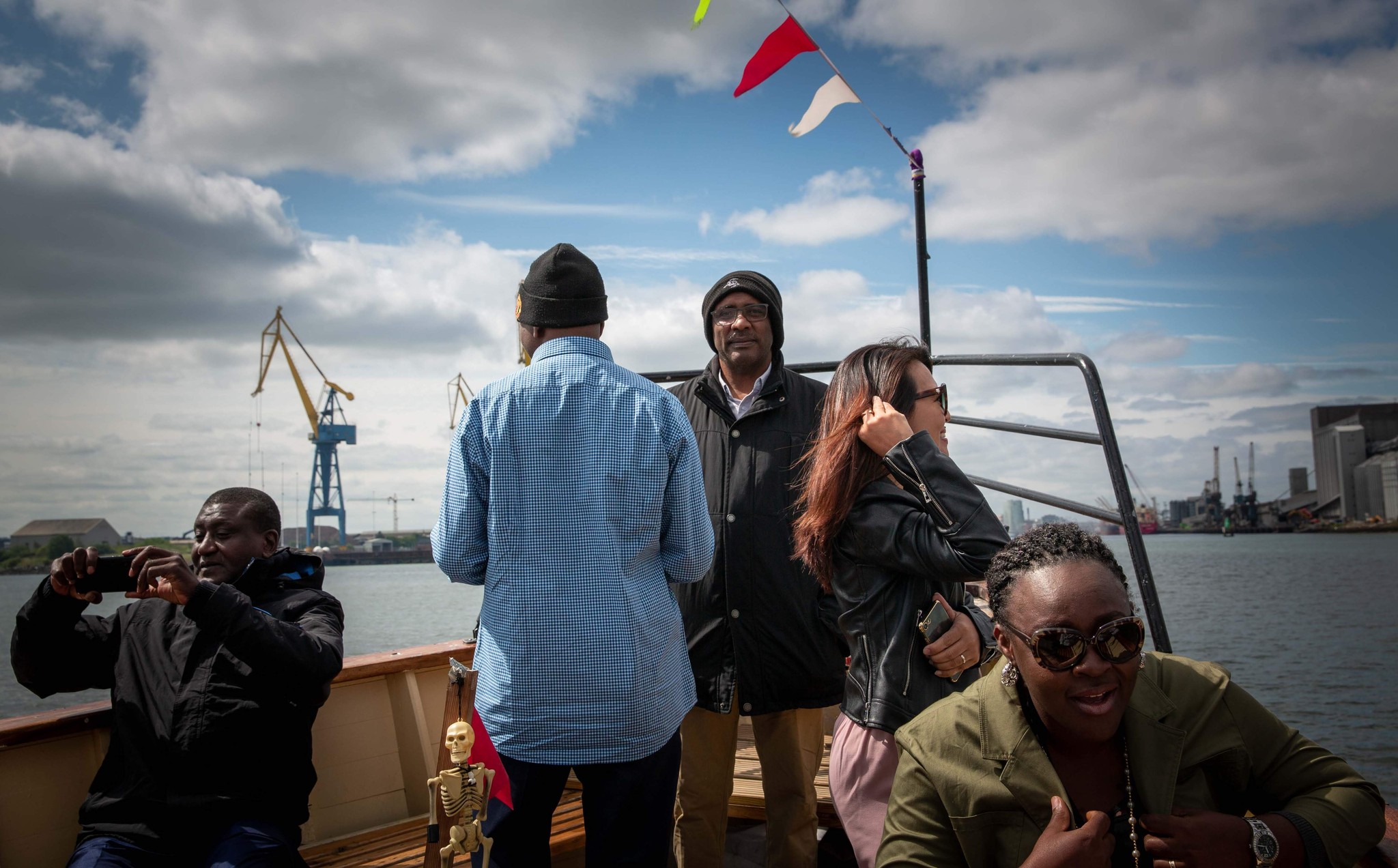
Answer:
[920,600,963,682]
[74,557,149,595]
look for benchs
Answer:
[299,720,840,868]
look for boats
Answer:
[2,349,1176,868]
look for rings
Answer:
[1169,859,1175,868]
[861,410,874,419]
[960,654,966,663]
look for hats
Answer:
[702,270,785,354]
[516,243,609,328]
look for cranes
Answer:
[250,305,357,553]
[1124,464,1159,521]
[1206,440,1259,523]
[345,493,415,533]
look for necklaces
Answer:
[1019,686,1141,868]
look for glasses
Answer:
[915,383,948,416]
[994,608,1145,671]
[710,304,769,326]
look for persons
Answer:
[10,488,344,868]
[796,342,1013,868]
[429,243,715,868]
[666,270,846,868]
[876,522,1385,868]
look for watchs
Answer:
[1241,815,1279,868]
